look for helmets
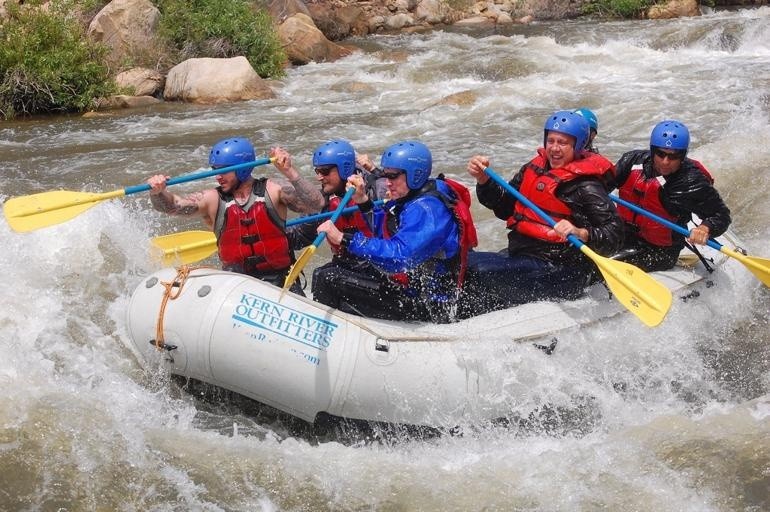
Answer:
[380,140,432,191]
[312,140,356,181]
[544,107,597,153]
[649,121,689,164]
[209,137,256,183]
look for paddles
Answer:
[608,193,770,289]
[3,155,276,233]
[282,174,360,290]
[481,164,673,328]
[150,191,391,268]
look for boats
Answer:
[125,212,761,441]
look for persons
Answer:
[146,138,325,297]
[573,107,601,155]
[463,111,625,312]
[285,138,391,261]
[311,141,478,324]
[587,120,732,287]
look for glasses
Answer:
[653,149,683,160]
[374,170,404,180]
[314,165,336,177]
